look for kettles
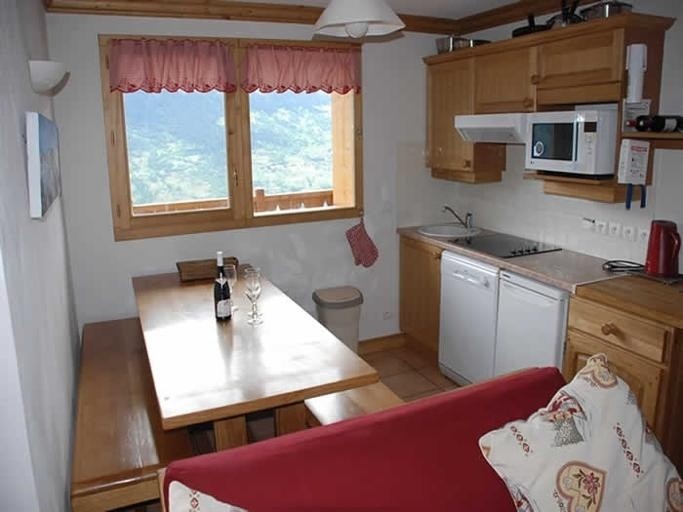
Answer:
[645,220,681,277]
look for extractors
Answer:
[455,112,527,144]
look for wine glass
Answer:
[224,263,263,325]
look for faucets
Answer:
[441,205,473,229]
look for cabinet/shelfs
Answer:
[470,37,575,113]
[560,294,682,477]
[420,47,505,186]
[534,13,674,113]
[393,224,445,364]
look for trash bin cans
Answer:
[312,286,363,353]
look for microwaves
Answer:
[525,110,617,180]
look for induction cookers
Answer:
[450,232,562,259]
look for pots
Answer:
[512,0,581,38]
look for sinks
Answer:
[418,225,481,238]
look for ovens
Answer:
[438,250,501,387]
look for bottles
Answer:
[214,251,232,321]
[626,115,683,132]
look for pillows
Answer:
[477,347,682,511]
[166,476,254,512]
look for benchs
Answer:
[155,359,568,512]
[299,353,404,432]
[68,311,172,511]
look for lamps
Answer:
[310,1,409,41]
[26,56,66,95]
